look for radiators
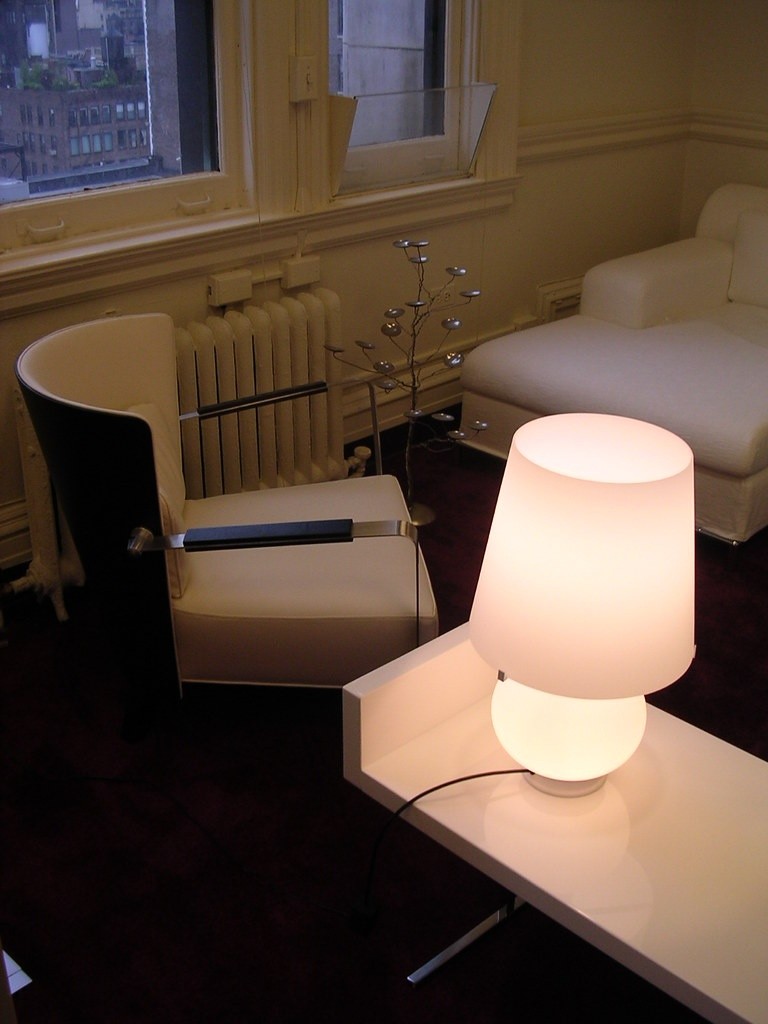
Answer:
[10,288,372,623]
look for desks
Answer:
[341,619,768,1024]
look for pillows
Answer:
[728,211,768,307]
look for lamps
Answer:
[469,414,696,800]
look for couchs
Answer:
[458,182,768,545]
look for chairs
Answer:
[18,313,438,703]
